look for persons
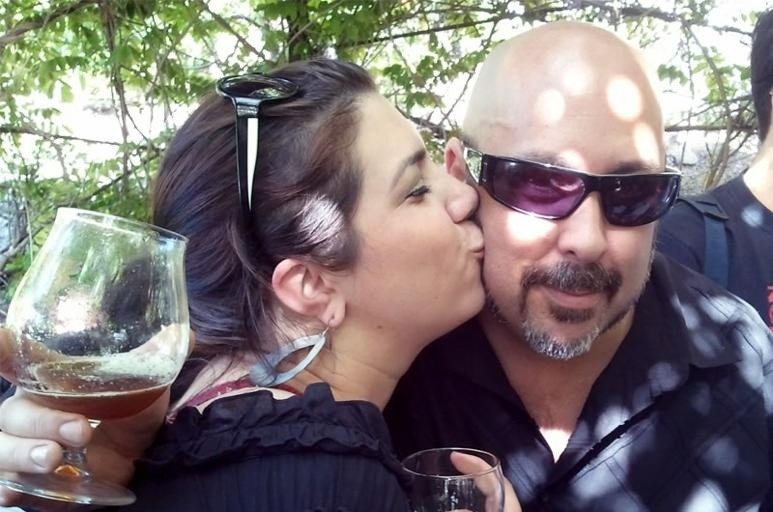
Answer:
[0,18,771,512]
[652,7,772,332]
[96,54,526,511]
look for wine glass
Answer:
[0,207,191,506]
[400,448,506,512]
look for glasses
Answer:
[463,147,683,226]
[216,71,300,228]
